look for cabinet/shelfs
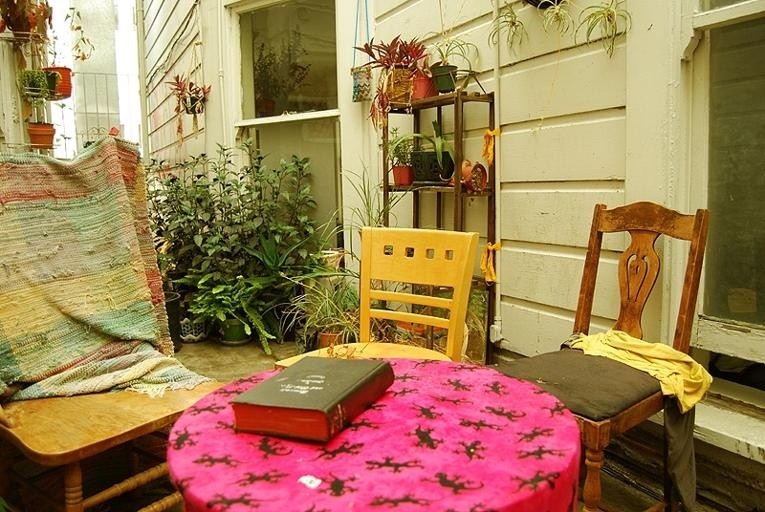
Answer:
[378,89,496,364]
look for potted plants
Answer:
[168,76,211,114]
[434,43,477,92]
[253,43,280,118]
[295,278,357,350]
[144,130,321,348]
[381,128,412,186]
[411,58,441,99]
[0,0,71,148]
[408,119,456,185]
[453,159,486,193]
[366,34,425,110]
[303,243,346,322]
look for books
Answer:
[228,355,394,442]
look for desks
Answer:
[167,357,582,512]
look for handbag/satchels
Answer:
[350,67,372,102]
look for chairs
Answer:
[0,137,226,512]
[492,202,711,512]
[274,225,479,368]
